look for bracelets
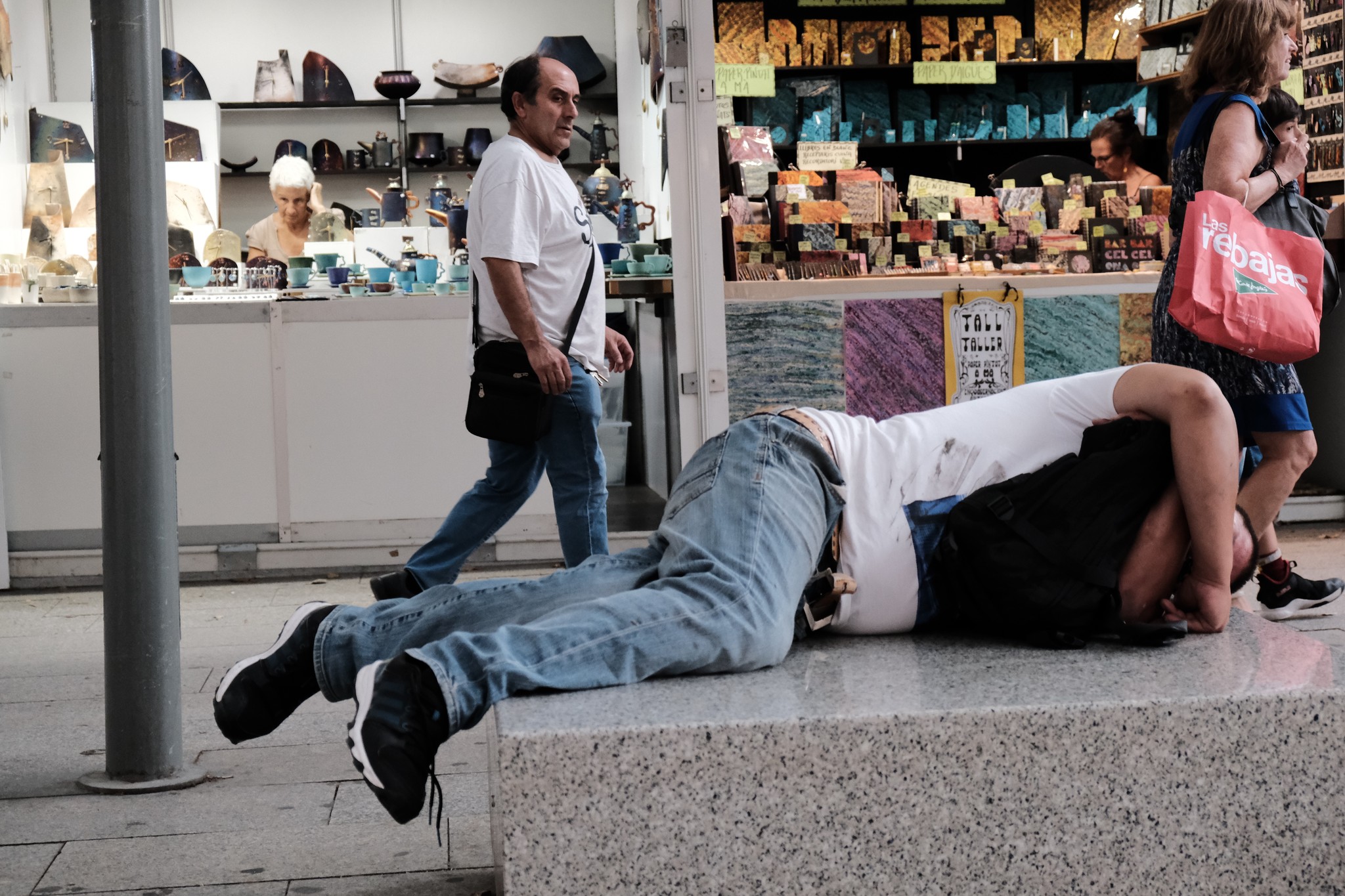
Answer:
[1269,166,1285,194]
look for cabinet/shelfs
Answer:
[174,87,633,254]
[717,12,1226,293]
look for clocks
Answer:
[28,107,96,164]
[22,161,217,233]
[202,229,242,263]
[162,47,211,100]
[163,120,204,162]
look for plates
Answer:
[445,280,468,282]
[316,274,328,277]
[349,273,366,276]
[286,286,311,288]
[404,292,434,296]
[452,291,469,294]
[334,293,352,297]
[368,292,394,296]
[328,284,339,288]
[604,265,672,277]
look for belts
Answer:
[743,404,856,631]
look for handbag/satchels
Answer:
[1167,177,1325,364]
[465,339,552,442]
[1201,89,1340,307]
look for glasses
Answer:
[1090,153,1114,165]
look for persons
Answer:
[244,153,354,269]
[213,361,1259,850]
[1090,118,1162,206]
[1149,0,1345,620]
[370,52,635,601]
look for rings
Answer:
[1303,145,1310,151]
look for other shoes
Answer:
[369,569,423,602]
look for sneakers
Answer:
[345,653,449,849]
[1251,560,1344,620]
[211,598,336,747]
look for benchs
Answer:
[436,609,1345,895]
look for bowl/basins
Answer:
[374,71,421,100]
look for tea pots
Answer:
[424,173,473,255]
[572,112,656,243]
[365,176,419,227]
[357,130,402,168]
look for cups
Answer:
[597,243,672,274]
[285,252,419,298]
[439,146,467,166]
[412,259,468,296]
[346,149,373,172]
[182,266,211,288]
